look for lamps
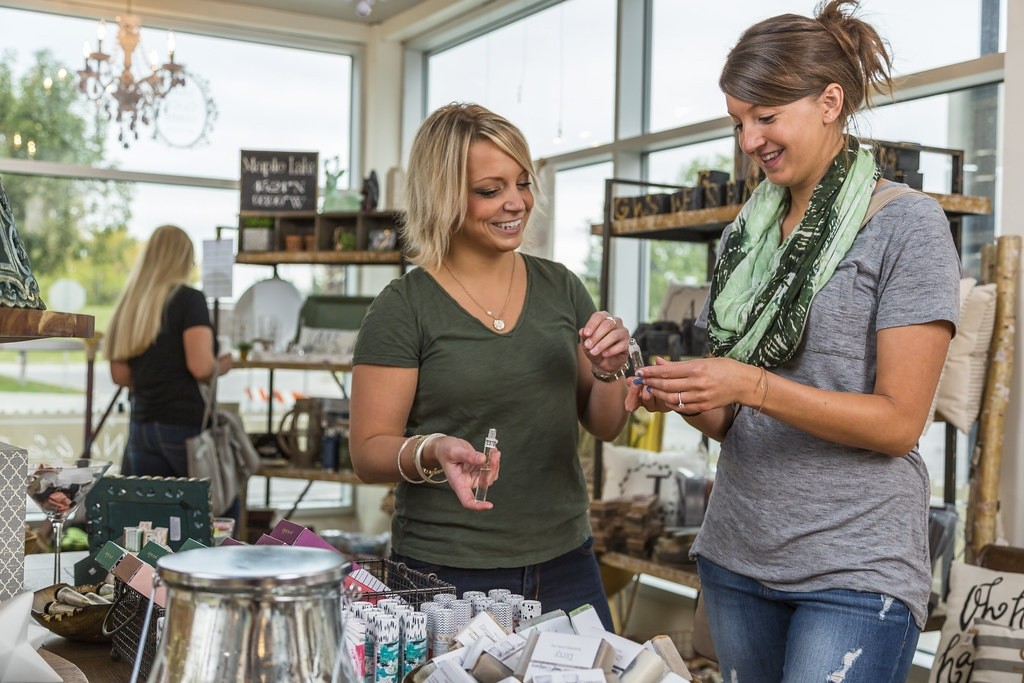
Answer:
[72,0,190,147]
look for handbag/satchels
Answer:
[184,359,261,517]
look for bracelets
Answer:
[752,366,769,418]
[398,433,448,484]
[674,410,701,417]
[591,363,629,382]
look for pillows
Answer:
[966,616,1024,683]
[928,559,1024,683]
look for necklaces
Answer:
[442,251,516,330]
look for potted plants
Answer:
[240,216,274,252]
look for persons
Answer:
[104,225,232,478]
[623,0,960,683]
[349,100,632,633]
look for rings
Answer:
[677,393,685,409]
[605,316,617,329]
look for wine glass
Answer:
[20,456,113,589]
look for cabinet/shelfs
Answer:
[590,136,993,632]
[200,209,423,538]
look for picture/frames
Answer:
[86,474,211,566]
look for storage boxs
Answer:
[94,519,415,614]
